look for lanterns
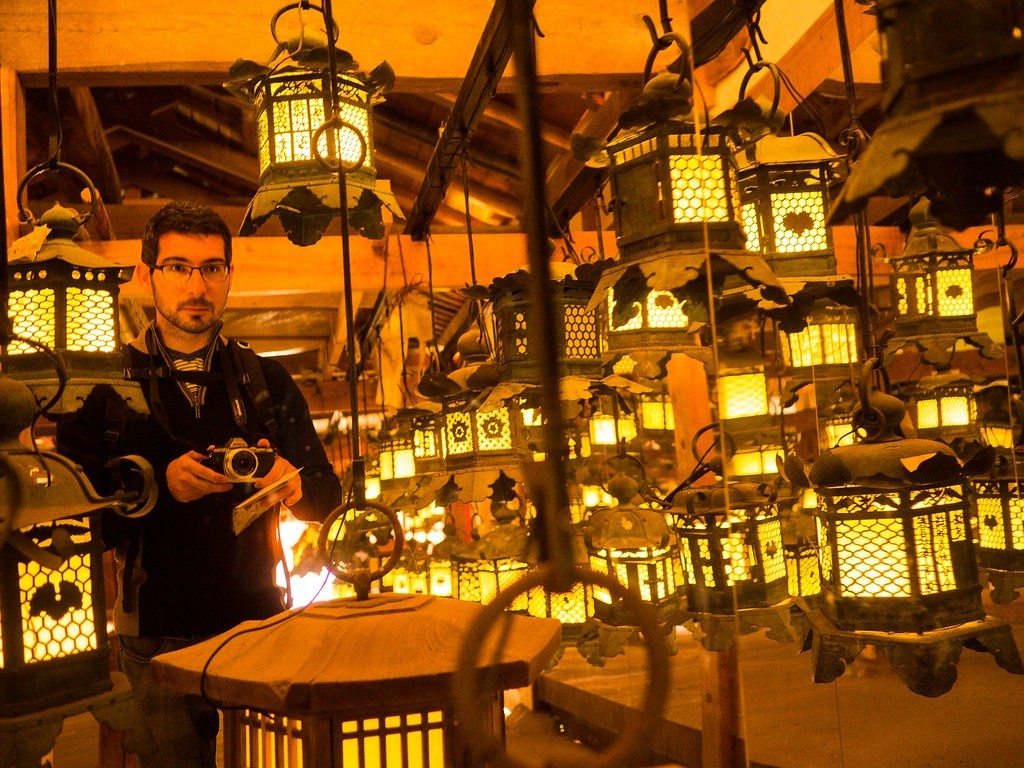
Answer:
[0,162,150,421]
[0,338,159,768]
[221,2,407,250]
[142,501,569,767]
[294,32,1024,694]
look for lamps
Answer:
[0,333,159,768]
[0,160,151,421]
[339,0,1024,695]
[152,498,561,768]
[222,1,406,246]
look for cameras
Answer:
[210,437,275,483]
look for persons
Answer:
[58,201,343,768]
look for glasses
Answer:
[142,259,230,283]
